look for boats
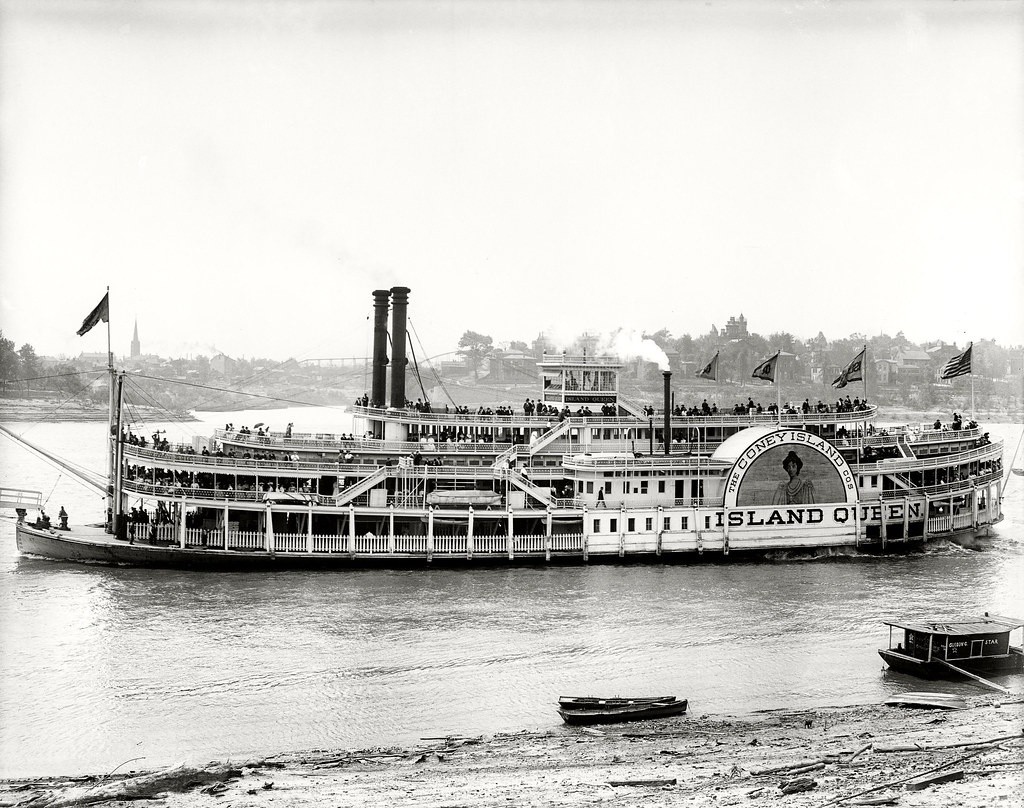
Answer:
[12,286,1010,560]
[556,698,688,726]
[878,610,1024,678]
[558,695,677,710]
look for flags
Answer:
[830,348,863,389]
[940,345,971,380]
[75,289,108,337]
[752,353,778,383]
[694,351,717,381]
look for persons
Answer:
[13,394,1004,551]
